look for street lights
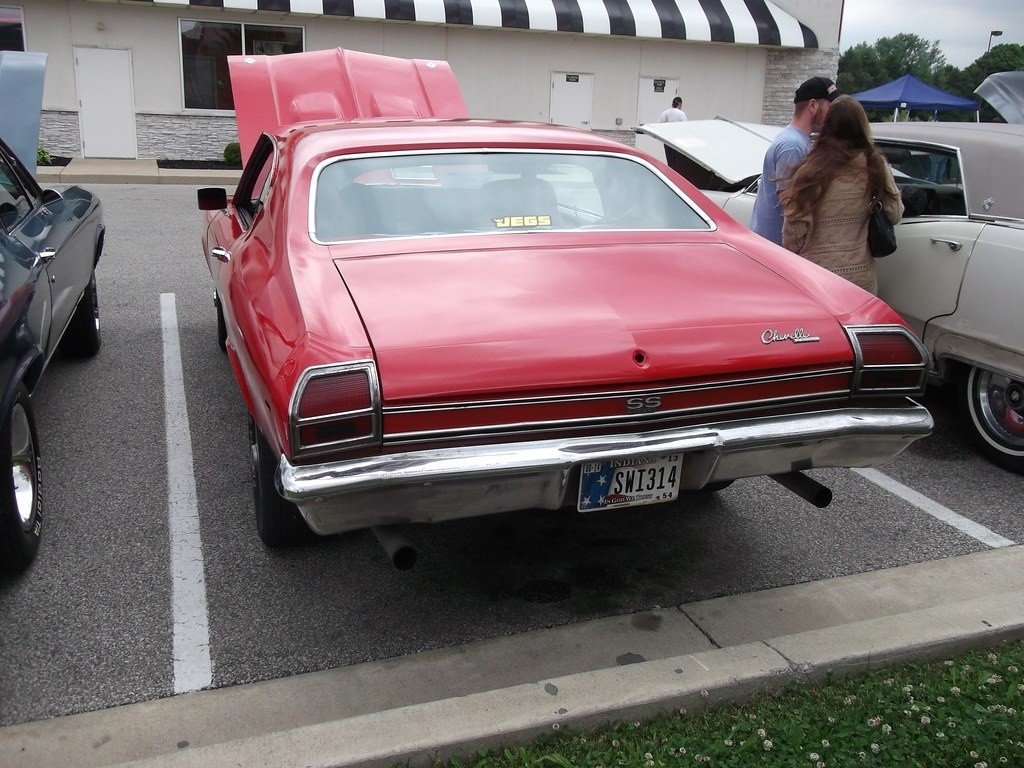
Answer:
[987,30,1003,52]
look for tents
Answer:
[851,73,980,123]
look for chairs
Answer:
[316,183,383,237]
[478,176,557,228]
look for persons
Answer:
[659,97,687,122]
[772,95,905,297]
[909,111,951,183]
[749,77,840,249]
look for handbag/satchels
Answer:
[866,154,899,258]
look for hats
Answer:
[794,76,840,103]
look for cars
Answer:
[1,50,106,574]
[639,119,1023,473]
[198,50,945,567]
